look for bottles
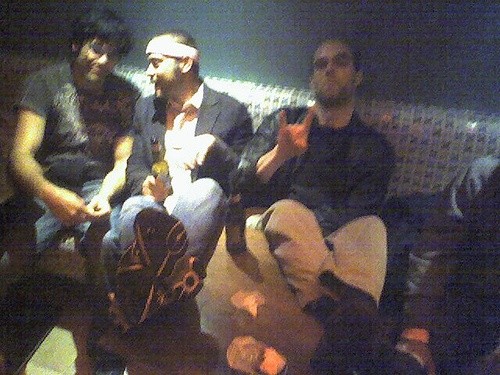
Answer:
[226,180,246,250]
[150,135,174,196]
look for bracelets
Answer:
[399,328,430,345]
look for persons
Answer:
[230,32,397,375]
[392,155,500,375]
[101,28,254,337]
[0,6,143,298]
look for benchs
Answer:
[0,50,500,205]
